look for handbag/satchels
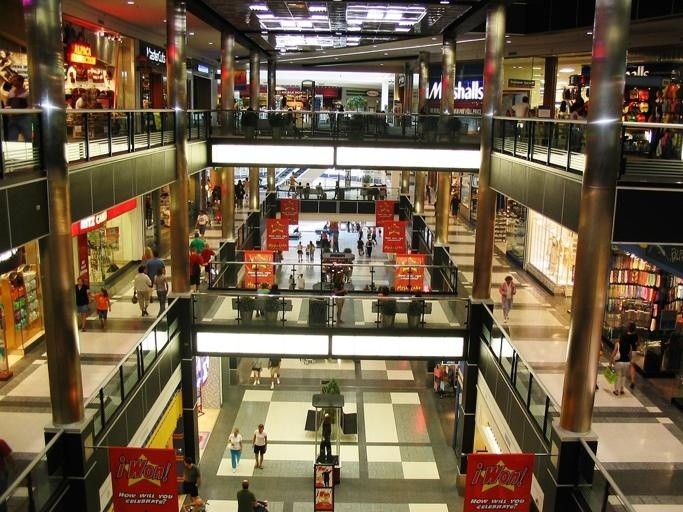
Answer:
[132,294,137,304]
[613,352,620,360]
[604,366,617,384]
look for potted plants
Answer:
[264,297,279,326]
[406,301,422,327]
[381,302,396,328]
[268,112,283,139]
[239,296,256,327]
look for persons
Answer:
[216,99,223,126]
[240,107,257,138]
[283,109,295,130]
[288,172,323,200]
[425,185,431,203]
[212,177,249,221]
[499,276,516,323]
[556,88,590,152]
[197,210,208,238]
[335,274,348,323]
[319,413,332,462]
[133,247,169,318]
[608,321,638,396]
[511,96,530,142]
[0,72,115,142]
[183,357,281,512]
[189,232,216,289]
[296,222,378,262]
[75,277,111,330]
[451,194,461,221]
[256,283,281,320]
[333,181,339,199]
[361,184,387,200]
[378,285,423,328]
[288,273,306,290]
[233,99,238,111]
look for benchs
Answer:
[232,298,293,321]
[372,301,432,324]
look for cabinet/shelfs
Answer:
[0,263,43,349]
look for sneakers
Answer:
[255,466,263,469]
[613,390,624,395]
[253,379,260,386]
[629,383,634,389]
[270,378,280,389]
[233,464,239,472]
